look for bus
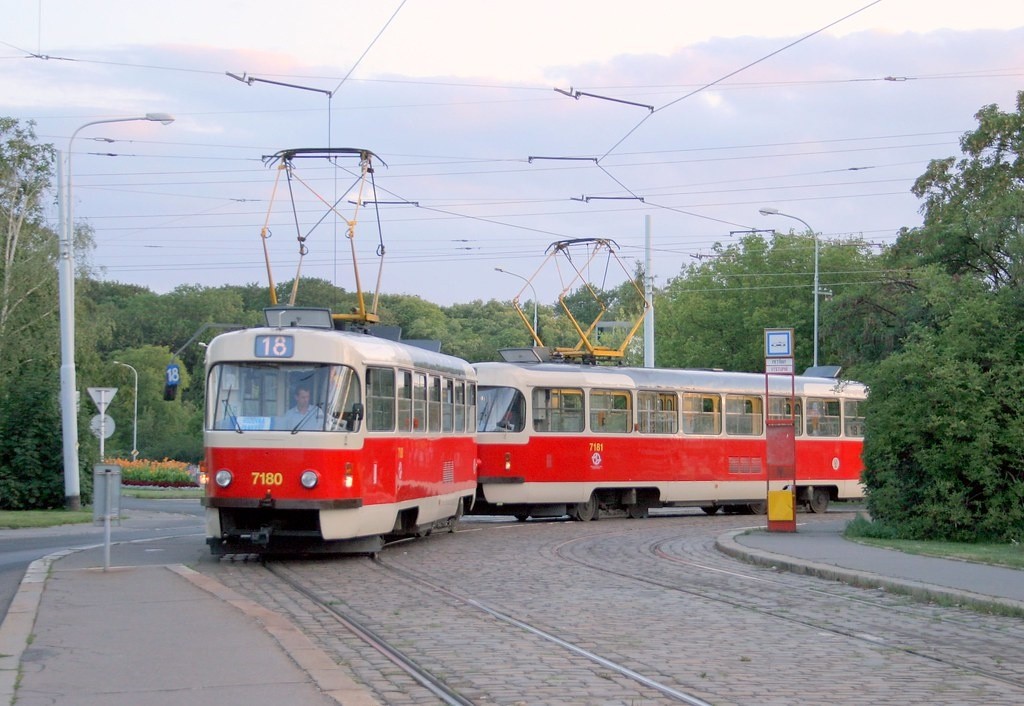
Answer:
[205,239,878,564]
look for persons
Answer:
[496,407,520,432]
[279,383,324,431]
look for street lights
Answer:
[62,112,174,514]
[495,268,539,348]
[111,360,140,465]
[759,204,820,368]
